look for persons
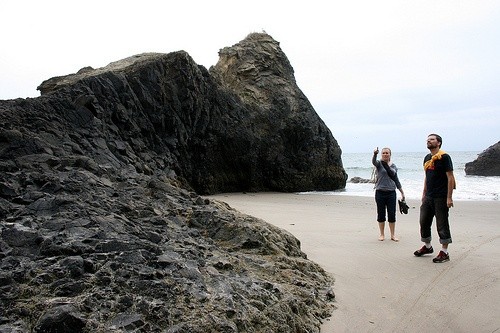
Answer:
[413,134,456,263]
[372,147,406,242]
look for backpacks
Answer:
[370,165,381,183]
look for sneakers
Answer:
[433,251,449,262]
[414,245,433,256]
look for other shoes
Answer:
[398,199,409,214]
